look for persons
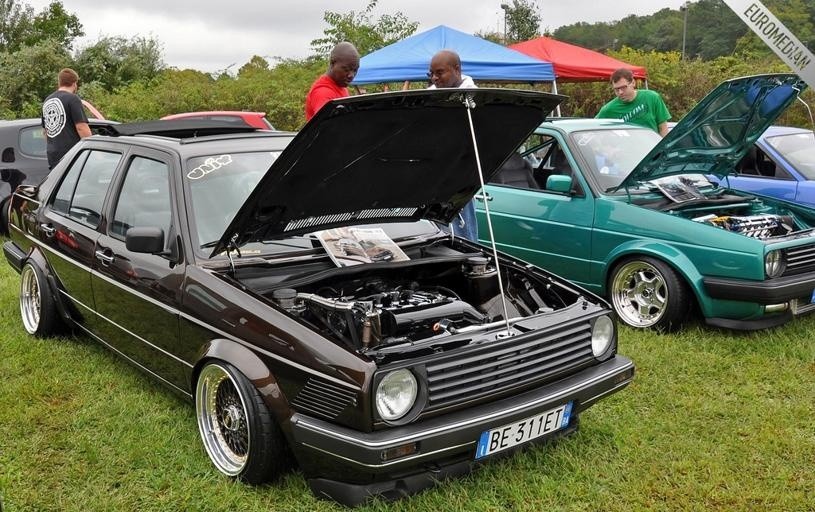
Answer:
[593,68,672,138]
[424,50,479,243]
[41,68,93,171]
[302,41,360,127]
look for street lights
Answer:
[500,3,510,48]
[680,5,690,62]
